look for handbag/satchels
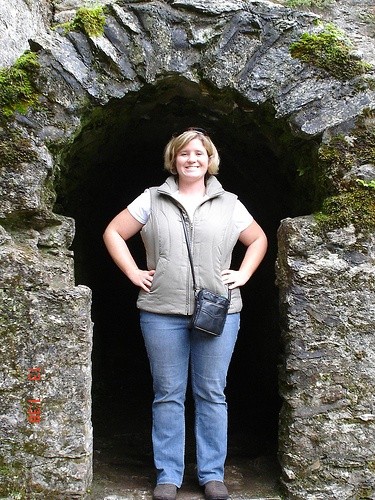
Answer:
[192,290,230,337]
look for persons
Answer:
[102,129,268,499]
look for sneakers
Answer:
[203,481,229,500]
[152,484,177,499]
